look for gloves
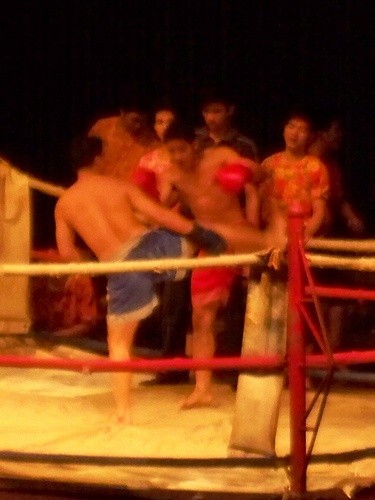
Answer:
[220,165,253,191]
[197,230,226,255]
[132,169,157,198]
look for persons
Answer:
[263,115,330,388]
[29,96,271,424]
[308,114,363,388]
